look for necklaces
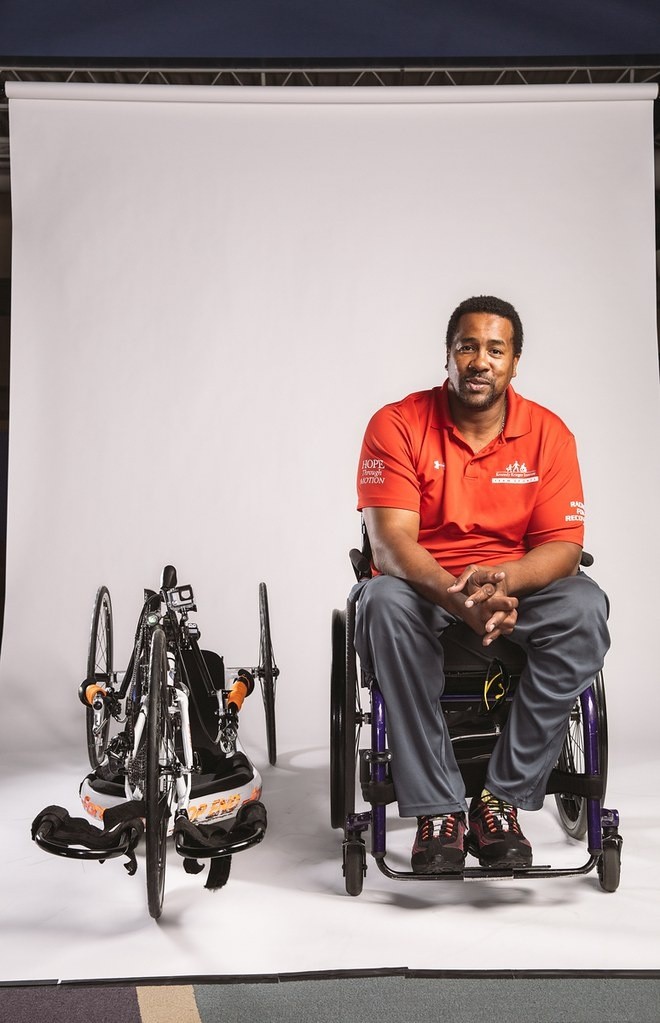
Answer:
[353,291,611,874]
[500,416,506,433]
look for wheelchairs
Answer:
[326,510,624,897]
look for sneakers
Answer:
[469,788,532,867]
[410,811,468,873]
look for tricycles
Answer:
[30,564,280,920]
